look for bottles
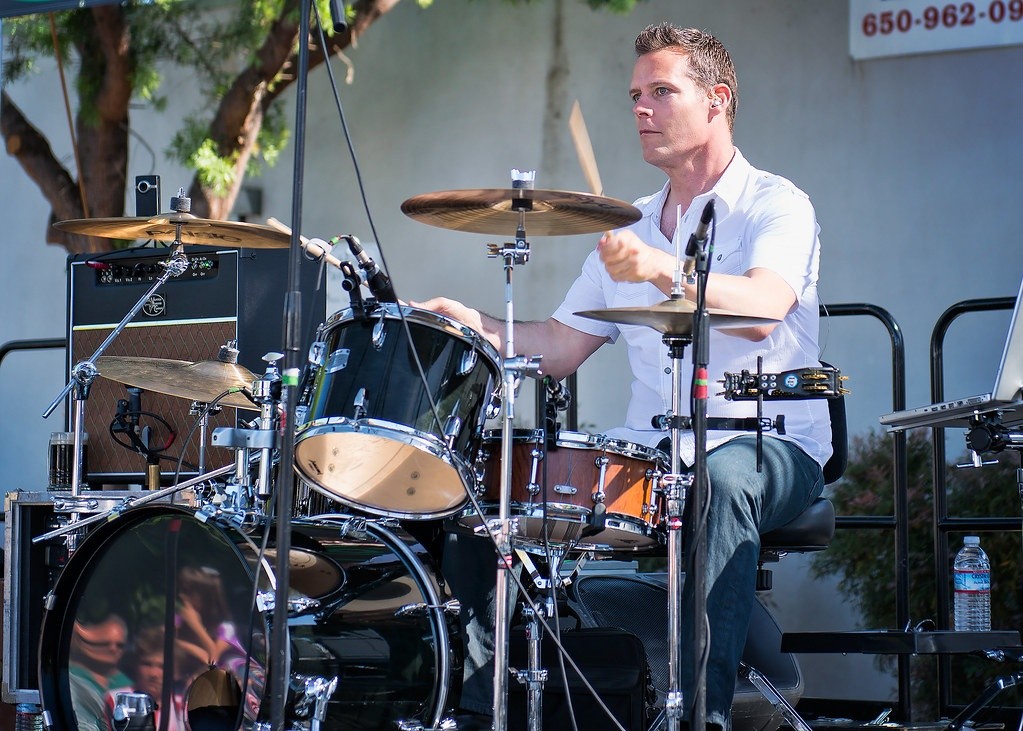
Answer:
[954,536,991,630]
[16,703,43,731]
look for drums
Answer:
[293,304,506,519]
[37,499,454,730]
[456,429,686,557]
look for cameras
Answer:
[136,175,161,216]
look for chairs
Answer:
[757,362,849,591]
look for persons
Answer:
[68,560,266,731]
[409,23,834,731]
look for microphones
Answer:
[347,235,398,304]
[545,375,572,411]
[685,199,717,261]
[241,386,283,423]
[123,382,144,437]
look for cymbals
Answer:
[573,299,782,343]
[396,187,644,237]
[715,367,851,402]
[49,214,306,250]
[86,352,289,412]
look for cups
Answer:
[47,432,91,490]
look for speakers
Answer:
[66,244,327,488]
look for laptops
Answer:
[879,281,1022,425]
